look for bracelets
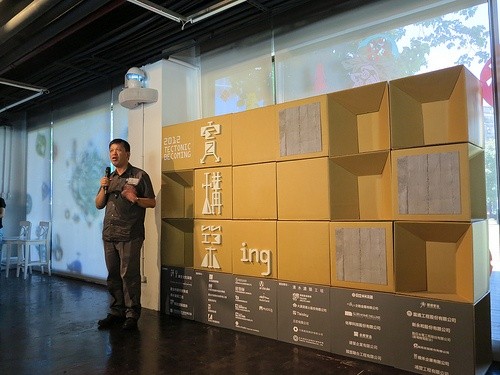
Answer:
[135,197,140,203]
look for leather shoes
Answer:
[98,314,125,326]
[123,317,137,329]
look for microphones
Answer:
[105,167,110,195]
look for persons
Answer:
[95,139,156,332]
[0,195,6,229]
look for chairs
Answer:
[0,220,52,280]
